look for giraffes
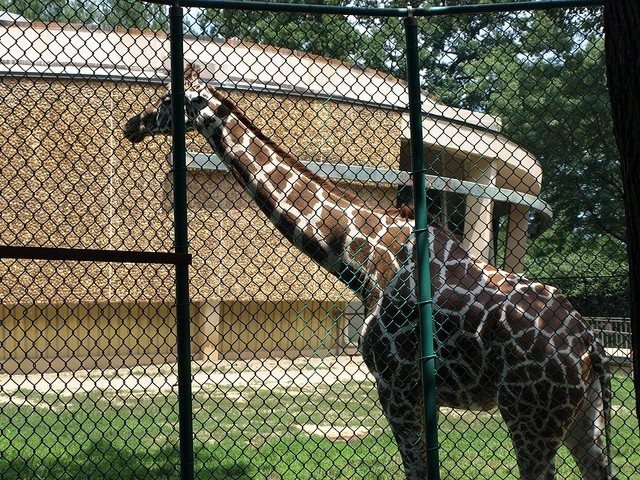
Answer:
[123,62,618,480]
